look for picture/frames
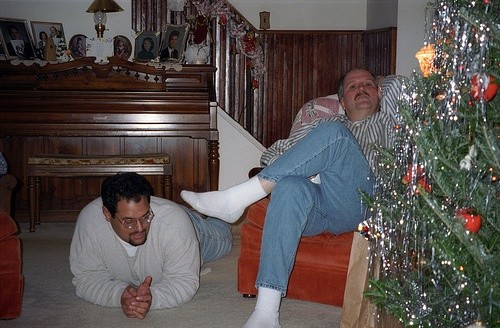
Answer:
[157,23,191,64]
[0,18,37,60]
[133,31,161,61]
[31,21,68,59]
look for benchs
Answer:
[28,154,172,232]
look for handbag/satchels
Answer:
[339,227,406,328]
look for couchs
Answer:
[238,95,354,309]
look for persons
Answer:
[180,69,416,328]
[137,37,156,59]
[15,42,25,56]
[115,39,127,58]
[48,25,63,51]
[38,30,49,55]
[68,170,235,320]
[159,31,180,60]
[8,25,21,40]
[74,36,85,57]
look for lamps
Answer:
[86,0,124,59]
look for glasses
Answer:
[115,206,155,229]
